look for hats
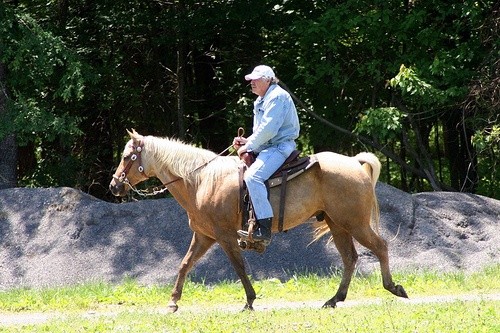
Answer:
[245,65,275,80]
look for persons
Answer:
[232,65,301,242]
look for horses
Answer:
[109,128,408,314]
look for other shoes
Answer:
[237,218,272,245]
[237,240,266,252]
